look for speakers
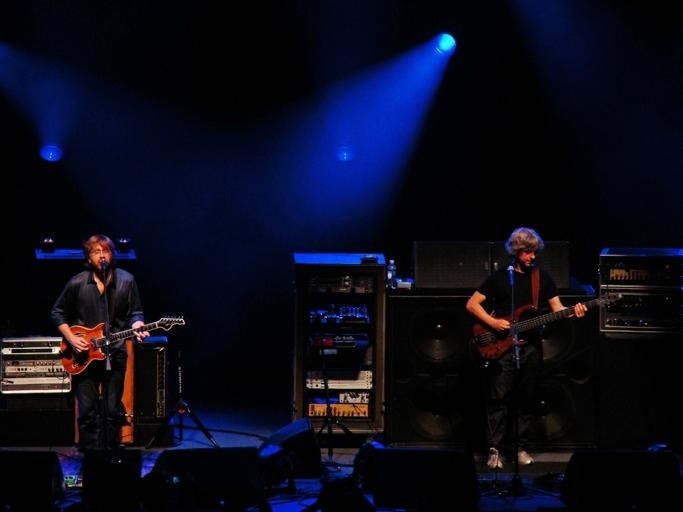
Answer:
[258,418,324,479]
[561,447,683,512]
[370,447,480,512]
[0,450,68,512]
[143,447,258,512]
[383,287,488,462]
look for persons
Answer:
[464,227,588,469]
[49,235,151,485]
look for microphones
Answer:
[100,259,108,271]
[507,266,515,288]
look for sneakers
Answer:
[517,447,535,467]
[482,447,504,472]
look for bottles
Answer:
[387,260,397,290]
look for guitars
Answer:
[472,291,621,360]
[58,315,186,377]
